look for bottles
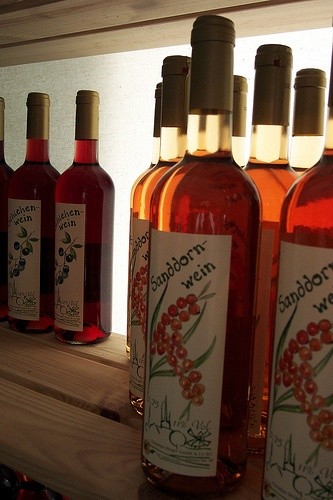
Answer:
[237,38,322,451]
[288,68,327,180]
[141,11,264,500]
[54,89,114,348]
[0,92,61,336]
[125,56,193,416]
[260,58,333,499]
